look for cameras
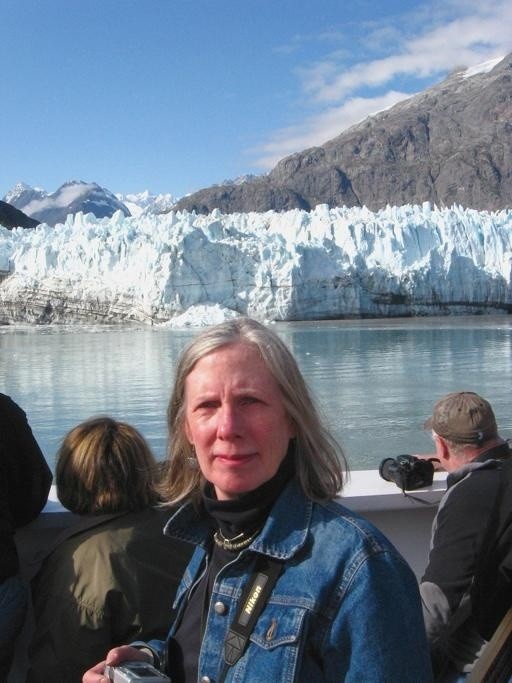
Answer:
[379,453,435,493]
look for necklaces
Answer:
[218,528,243,544]
[212,524,264,551]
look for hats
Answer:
[424,391,497,443]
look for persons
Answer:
[399,390,512,683]
[0,392,53,578]
[79,314,433,683]
[31,418,198,683]
